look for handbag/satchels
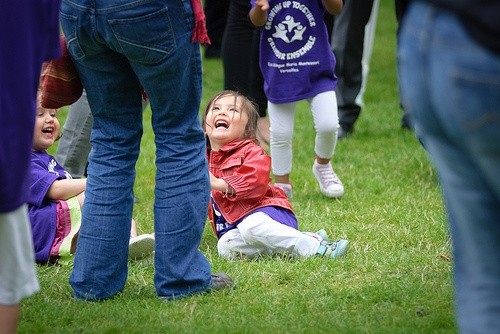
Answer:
[41,35,85,108]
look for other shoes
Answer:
[338,126,349,141]
[327,240,349,258]
[127,234,156,260]
[316,229,329,244]
[208,273,235,292]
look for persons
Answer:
[0,0,62,334]
[59,0,235,305]
[245,0,346,204]
[394,1,499,333]
[52,79,152,204]
[199,0,421,137]
[200,90,349,261]
[23,87,158,267]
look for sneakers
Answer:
[312,158,345,197]
[274,182,291,199]
